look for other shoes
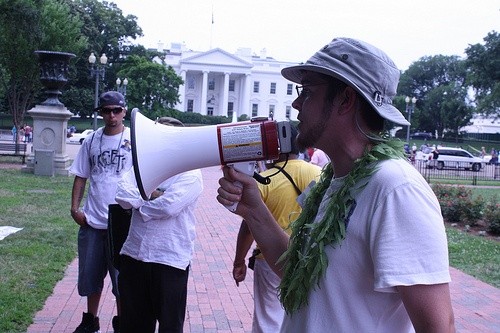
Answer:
[72,311,100,333]
[111,315,117,333]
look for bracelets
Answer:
[71,210,79,215]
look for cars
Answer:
[422,147,487,172]
[70,129,94,144]
[410,128,433,141]
[380,126,404,139]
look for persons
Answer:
[234,161,264,175]
[216,38,456,333]
[233,153,324,333]
[298,148,331,169]
[68,90,203,333]
[403,141,444,169]
[480,145,499,164]
[67,124,78,137]
[457,145,463,149]
[11,124,33,143]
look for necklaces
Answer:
[100,125,124,166]
[273,135,412,320]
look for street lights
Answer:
[89,53,107,131]
[404,96,417,144]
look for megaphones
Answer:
[130,108,299,213]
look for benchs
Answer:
[0,143,27,165]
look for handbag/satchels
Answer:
[107,203,134,268]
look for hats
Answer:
[281,37,410,131]
[94,90,126,111]
[154,116,184,129]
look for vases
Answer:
[36,50,77,106]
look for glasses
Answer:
[101,107,124,114]
[295,80,331,95]
